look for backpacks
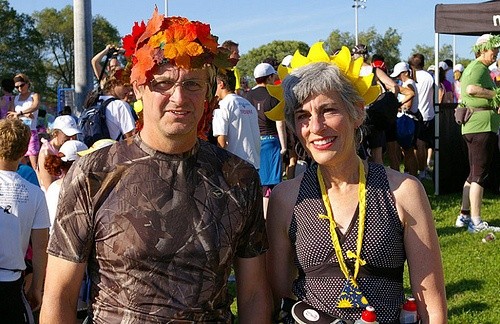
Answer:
[77,98,122,148]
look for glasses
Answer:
[15,84,26,88]
[241,82,246,84]
[142,78,208,92]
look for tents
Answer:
[434,0,500,194]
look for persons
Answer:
[243,57,311,222]
[407,53,434,177]
[90,45,140,120]
[437,58,465,104]
[457,34,500,232]
[221,40,240,95]
[390,61,419,177]
[334,44,414,172]
[37,4,274,324]
[426,65,447,166]
[0,74,40,186]
[263,42,447,324]
[0,115,56,324]
[23,115,88,324]
[213,68,261,171]
[76,66,137,158]
[488,51,500,88]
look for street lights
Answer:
[352,0,367,45]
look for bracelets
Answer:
[20,111,23,115]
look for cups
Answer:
[482,233,496,244]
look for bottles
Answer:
[399,296,418,324]
[354,305,380,324]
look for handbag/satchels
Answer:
[455,106,474,124]
[282,297,354,324]
[366,67,399,143]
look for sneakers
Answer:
[467,221,500,233]
[454,216,472,228]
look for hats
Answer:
[453,64,464,73]
[476,34,500,48]
[438,61,449,69]
[426,65,435,72]
[52,114,83,136]
[76,139,118,157]
[390,61,410,77]
[254,63,279,78]
[282,55,293,68]
[59,140,89,162]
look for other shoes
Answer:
[419,171,427,180]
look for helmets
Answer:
[353,44,368,54]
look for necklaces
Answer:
[314,159,372,307]
